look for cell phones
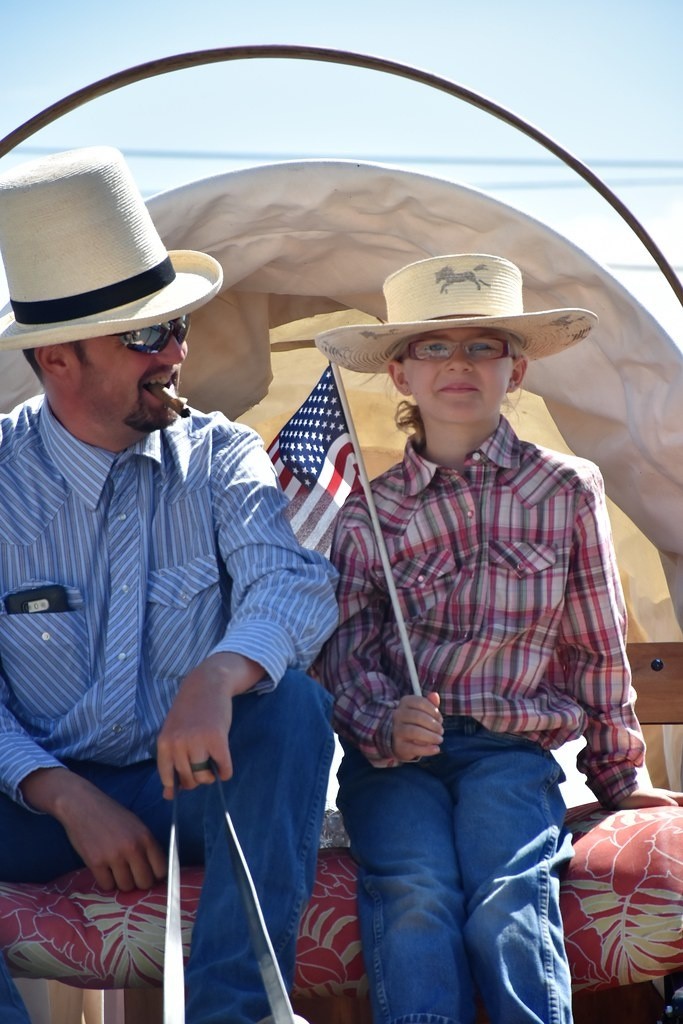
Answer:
[5,585,69,615]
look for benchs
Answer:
[0,639,683,1024]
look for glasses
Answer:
[111,313,191,354]
[397,338,519,361]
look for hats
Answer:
[0,147,224,352]
[314,254,598,374]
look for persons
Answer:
[311,258,683,1024]
[0,273,338,1024]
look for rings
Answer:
[191,761,210,772]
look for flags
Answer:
[266,367,366,562]
